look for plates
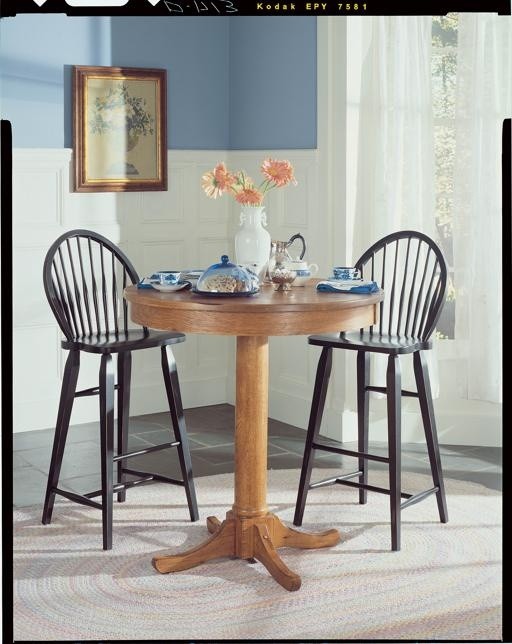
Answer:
[190,287,259,298]
[150,282,189,293]
[328,279,363,286]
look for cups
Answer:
[333,267,359,283]
[158,270,181,285]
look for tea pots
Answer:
[290,257,319,287]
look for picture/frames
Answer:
[71,65,168,194]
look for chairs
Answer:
[292,229,447,550]
[41,228,199,550]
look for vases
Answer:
[199,204,298,275]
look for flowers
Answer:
[200,158,300,205]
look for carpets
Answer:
[13,467,503,644]
